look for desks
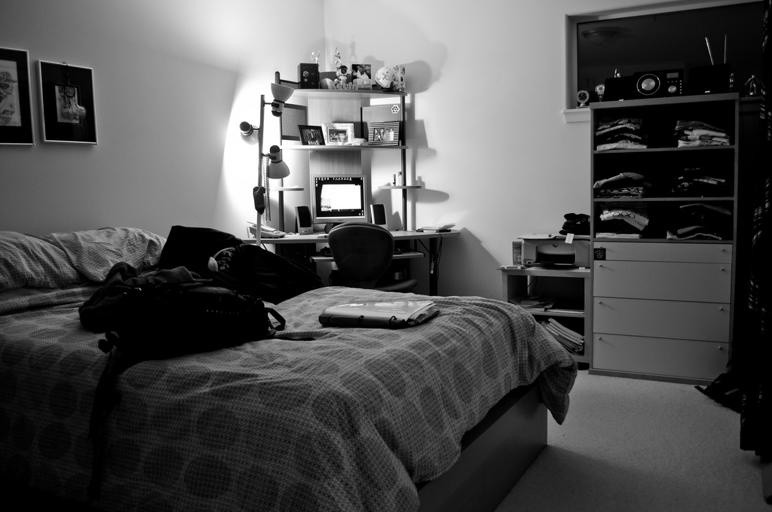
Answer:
[239,226,459,296]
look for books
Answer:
[319,298,440,329]
[518,298,584,354]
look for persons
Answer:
[352,66,361,78]
[307,132,319,145]
[355,68,370,85]
[374,129,395,142]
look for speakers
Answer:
[295,206,314,237]
[370,204,387,233]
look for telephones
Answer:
[248,218,287,238]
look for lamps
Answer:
[237,81,296,251]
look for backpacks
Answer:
[96,284,285,412]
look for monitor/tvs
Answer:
[313,175,368,227]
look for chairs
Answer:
[325,223,420,291]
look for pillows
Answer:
[1,223,174,290]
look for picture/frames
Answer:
[298,121,400,147]
[0,47,98,147]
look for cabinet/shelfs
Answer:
[587,91,741,384]
[274,83,422,192]
[499,263,590,364]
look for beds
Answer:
[1,280,552,512]
